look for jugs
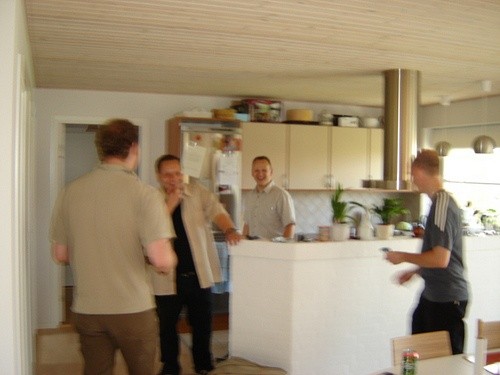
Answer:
[437,141,452,156]
[474,135,495,153]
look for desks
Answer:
[370,347,500,375]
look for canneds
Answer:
[401,347,419,375]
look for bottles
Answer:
[357,210,374,239]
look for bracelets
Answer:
[224,228,236,236]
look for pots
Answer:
[210,109,237,121]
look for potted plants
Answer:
[368,195,411,240]
[329,183,366,241]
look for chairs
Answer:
[392,331,453,366]
[477,318,500,349]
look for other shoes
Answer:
[195,365,213,375]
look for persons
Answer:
[147,154,241,375]
[241,156,296,240]
[52,118,177,375]
[386,150,469,356]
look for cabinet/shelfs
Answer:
[164,116,387,192]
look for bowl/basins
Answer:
[338,117,358,127]
[397,221,413,230]
